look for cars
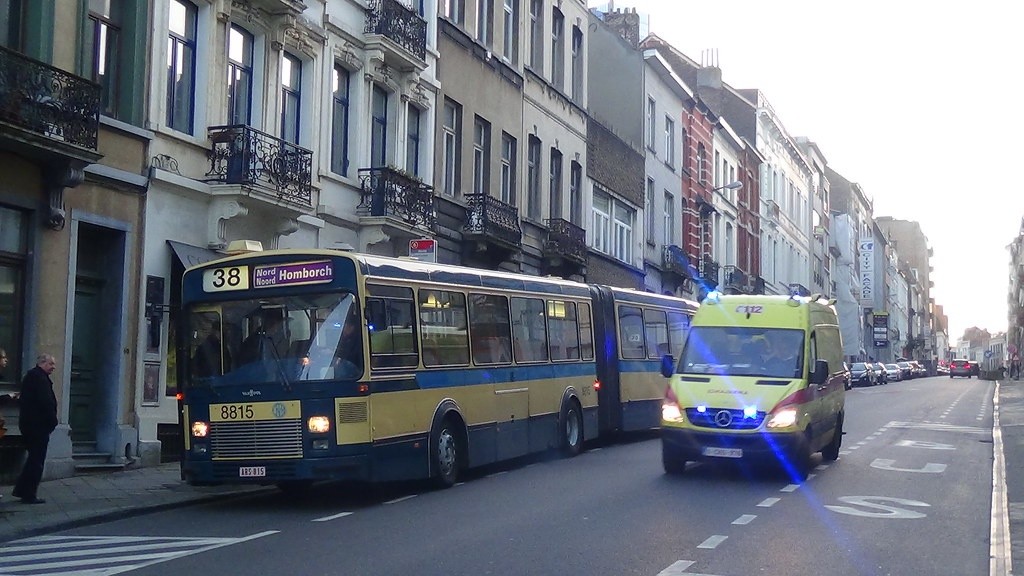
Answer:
[885,364,903,381]
[911,361,928,379]
[968,360,980,376]
[849,362,878,387]
[842,362,852,391]
[873,362,889,384]
[898,361,915,380]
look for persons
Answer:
[774,336,801,377]
[12,353,59,505]
[321,311,363,364]
[193,319,238,377]
[238,311,290,364]
[0,348,8,437]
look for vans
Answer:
[660,291,848,485]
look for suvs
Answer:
[949,359,972,378]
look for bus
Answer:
[172,239,703,484]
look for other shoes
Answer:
[11,492,22,498]
[22,497,45,504]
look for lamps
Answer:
[713,182,743,191]
